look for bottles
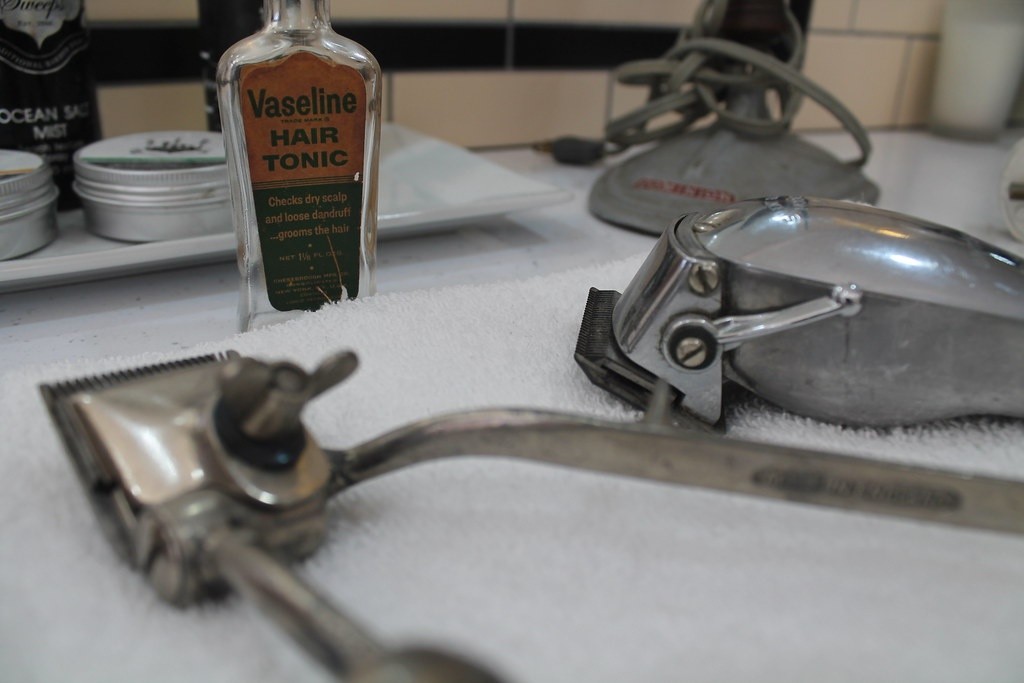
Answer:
[216,0,381,333]
[0,0,104,212]
